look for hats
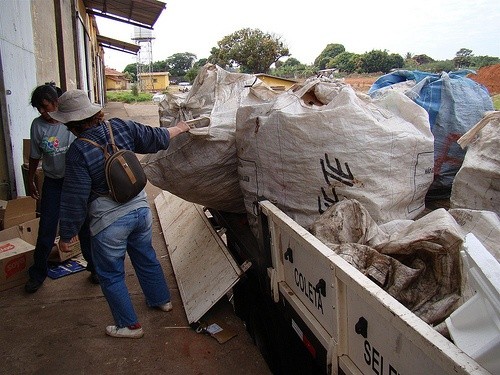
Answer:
[47,89,102,124]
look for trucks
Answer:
[154,78,499,375]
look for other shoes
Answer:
[90,272,100,284]
[24,278,42,294]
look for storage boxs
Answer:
[0,196,82,291]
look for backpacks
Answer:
[77,120,147,206]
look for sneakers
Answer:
[158,302,173,311]
[106,325,144,338]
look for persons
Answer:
[22,80,77,295]
[46,89,191,339]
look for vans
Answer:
[179,83,191,92]
